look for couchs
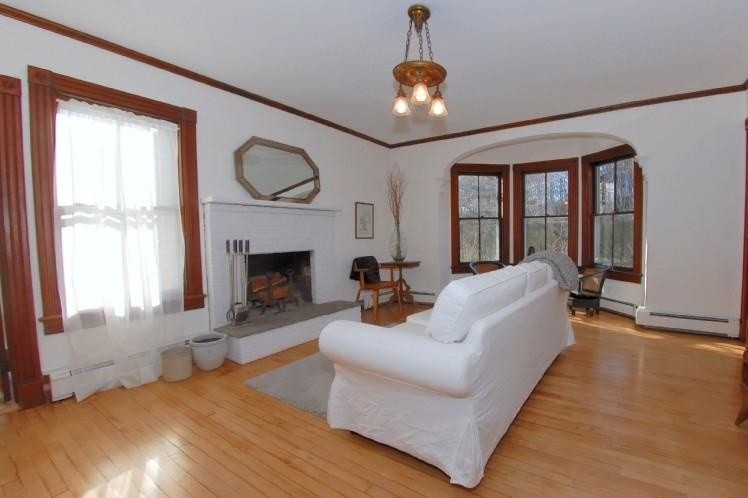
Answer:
[318,260,576,489]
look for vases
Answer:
[390,223,407,260]
[189,333,228,371]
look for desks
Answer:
[378,261,421,304]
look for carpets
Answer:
[244,322,399,419]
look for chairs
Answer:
[349,255,402,320]
[469,260,506,274]
[567,263,612,316]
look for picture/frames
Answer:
[355,202,374,238]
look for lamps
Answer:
[392,4,449,118]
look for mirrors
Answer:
[234,136,321,204]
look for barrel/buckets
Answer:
[162,347,192,383]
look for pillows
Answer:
[516,262,549,294]
[428,265,527,344]
